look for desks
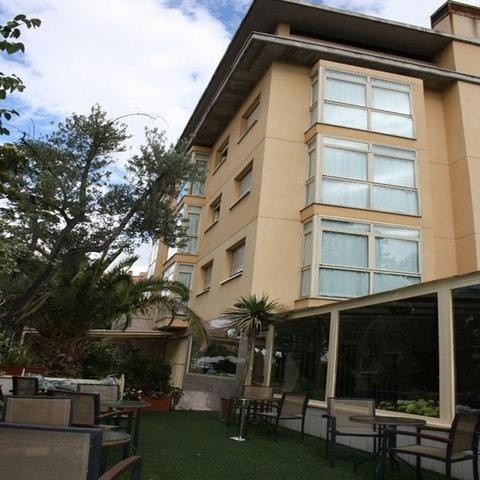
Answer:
[347,412,426,479]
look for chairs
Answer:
[0,374,153,479]
[321,396,381,463]
[224,384,311,443]
[388,408,480,479]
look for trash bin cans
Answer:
[218,396,235,423]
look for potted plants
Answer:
[141,380,185,413]
[0,353,29,377]
